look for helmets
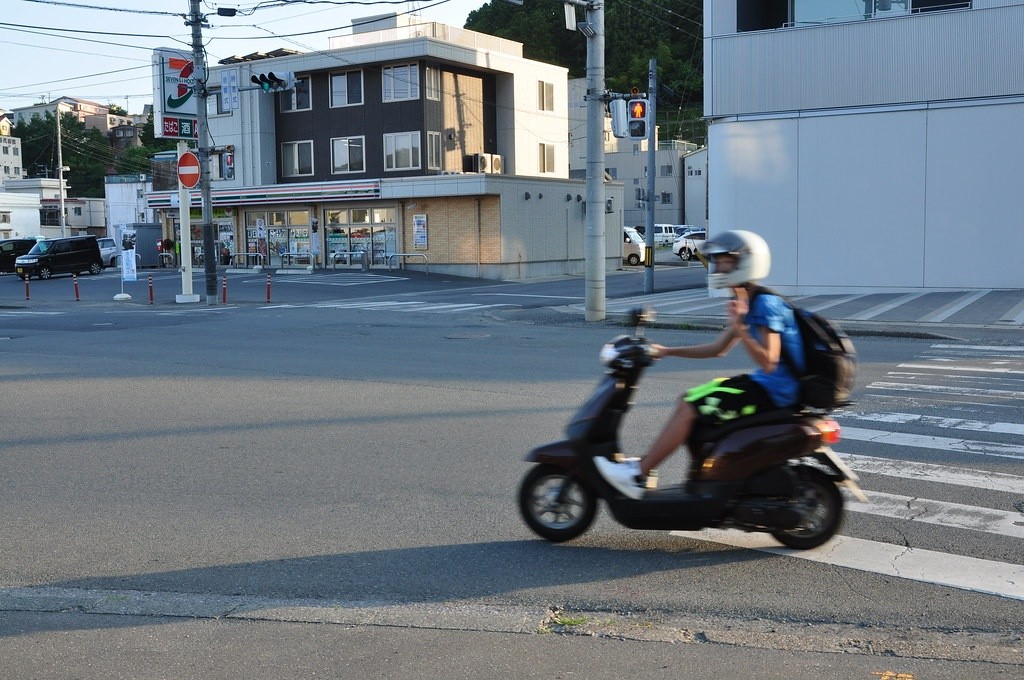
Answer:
[697,229,772,289]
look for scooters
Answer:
[514,307,870,551]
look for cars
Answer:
[97,236,116,269]
[623,225,646,267]
[672,230,706,261]
[633,223,706,247]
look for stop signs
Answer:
[176,152,202,188]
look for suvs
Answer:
[0,237,37,273]
[15,234,102,281]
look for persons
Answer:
[594,230,806,500]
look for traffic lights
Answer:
[251,70,296,94]
[607,99,628,139]
[627,98,650,141]
[221,152,236,181]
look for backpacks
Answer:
[795,307,857,408]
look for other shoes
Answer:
[615,452,659,488]
[592,456,645,500]
